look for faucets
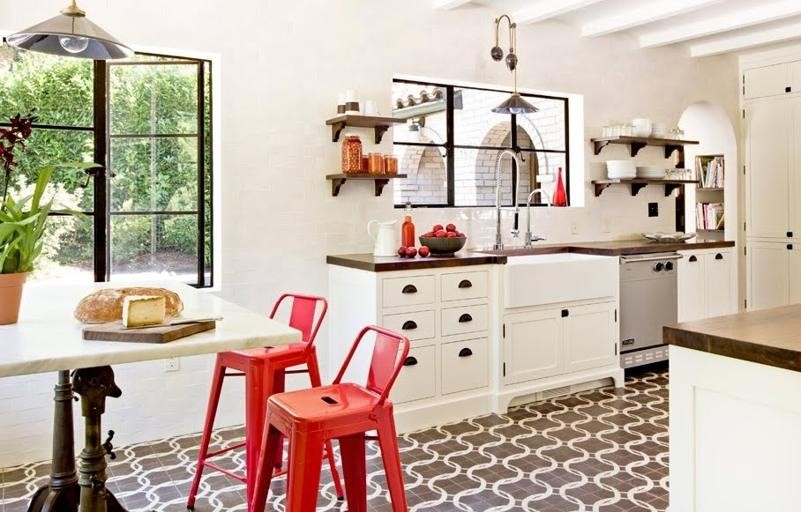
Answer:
[494,149,520,250]
[524,189,551,248]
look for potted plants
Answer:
[1,157,104,325]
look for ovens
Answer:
[616,252,678,368]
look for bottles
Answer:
[402,215,415,249]
[552,167,567,207]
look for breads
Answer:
[75,286,183,322]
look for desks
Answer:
[662,302,799,512]
[572,239,738,374]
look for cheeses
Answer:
[123,294,165,328]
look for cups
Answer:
[336,95,381,117]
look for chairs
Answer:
[180,291,344,510]
[249,325,410,512]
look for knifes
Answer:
[120,315,225,329]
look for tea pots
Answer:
[366,219,400,257]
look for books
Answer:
[697,156,726,190]
[697,201,725,229]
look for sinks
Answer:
[504,253,619,308]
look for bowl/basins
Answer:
[600,118,666,138]
[419,237,467,258]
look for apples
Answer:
[398,247,417,258]
[422,224,465,238]
[419,246,429,257]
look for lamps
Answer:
[3,2,134,72]
[489,14,538,119]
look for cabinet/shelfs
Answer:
[591,132,701,192]
[678,251,737,322]
[500,301,625,388]
[325,103,407,196]
[380,252,492,438]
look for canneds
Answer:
[342,133,398,174]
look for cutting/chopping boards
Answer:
[82,318,216,344]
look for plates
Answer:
[641,231,695,242]
[605,159,664,180]
